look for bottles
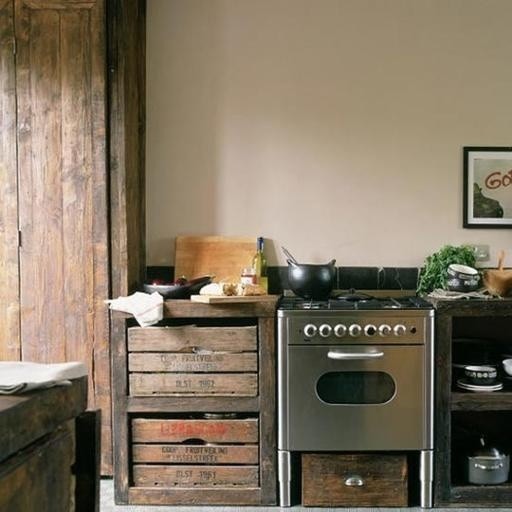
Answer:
[253,237,268,296]
[241,268,256,287]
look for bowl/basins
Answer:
[464,366,496,385]
[445,263,480,291]
[142,276,213,300]
[502,358,511,376]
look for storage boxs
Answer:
[297,454,410,507]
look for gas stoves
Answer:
[278,296,434,347]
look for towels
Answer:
[106,289,165,329]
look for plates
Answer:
[457,379,504,394]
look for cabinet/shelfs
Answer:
[445,294,512,511]
[118,292,278,506]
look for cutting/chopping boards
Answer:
[190,294,278,306]
[173,234,264,290]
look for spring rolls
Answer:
[199,283,238,296]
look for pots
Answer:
[285,258,338,299]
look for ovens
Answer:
[276,346,432,453]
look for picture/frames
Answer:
[460,144,512,230]
